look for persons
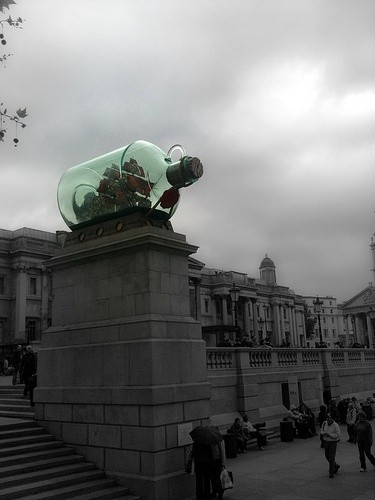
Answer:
[351,397,366,420]
[370,393,375,418]
[318,397,350,428]
[19,345,33,380]
[318,342,345,348]
[187,443,227,500]
[287,402,318,439]
[21,350,37,407]
[346,403,356,443]
[320,411,341,478]
[241,415,265,450]
[354,412,375,472]
[12,345,24,385]
[217,335,291,347]
[230,418,248,452]
[2,358,8,376]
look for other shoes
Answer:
[260,447,264,449]
[333,465,340,474]
[13,382,16,385]
[347,440,349,442]
[371,465,375,471]
[244,450,248,453]
[17,382,23,384]
[329,471,334,478]
[360,469,366,472]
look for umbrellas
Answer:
[189,426,224,450]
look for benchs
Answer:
[226,422,275,452]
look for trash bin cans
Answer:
[223,435,237,458]
[280,421,293,442]
[362,406,373,420]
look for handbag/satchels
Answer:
[320,434,324,448]
[220,466,233,489]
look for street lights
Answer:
[227,280,244,347]
[313,297,328,348]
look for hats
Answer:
[358,411,366,419]
[290,405,296,411]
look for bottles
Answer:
[57,139,203,232]
